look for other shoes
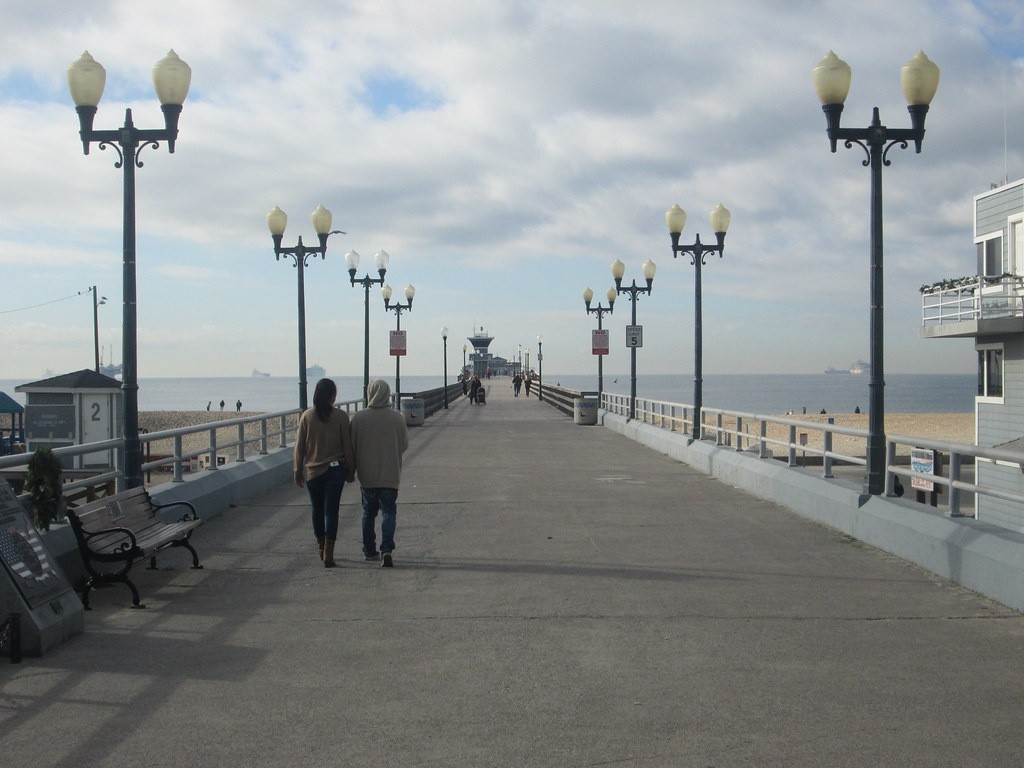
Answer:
[365,551,379,561]
[381,552,393,567]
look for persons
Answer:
[207,401,211,411]
[219,400,225,411]
[512,373,522,394]
[236,400,242,413]
[467,376,481,405]
[350,379,408,567]
[460,376,468,396]
[293,378,357,569]
[524,378,532,397]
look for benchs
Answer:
[67,485,204,608]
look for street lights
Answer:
[665,201,733,436]
[63,48,192,491]
[440,325,449,409]
[811,45,943,497]
[518,345,523,381]
[610,257,657,418]
[536,333,543,401]
[583,287,617,408]
[265,203,336,410]
[343,246,391,409]
[382,283,415,411]
[461,343,468,396]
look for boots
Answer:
[316,536,324,560]
[323,536,336,567]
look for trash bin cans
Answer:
[400,399,425,426]
[574,398,598,426]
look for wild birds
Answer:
[557,382,560,386]
[614,379,617,383]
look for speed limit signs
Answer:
[626,323,644,349]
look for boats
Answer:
[822,366,852,374]
[848,361,869,373]
